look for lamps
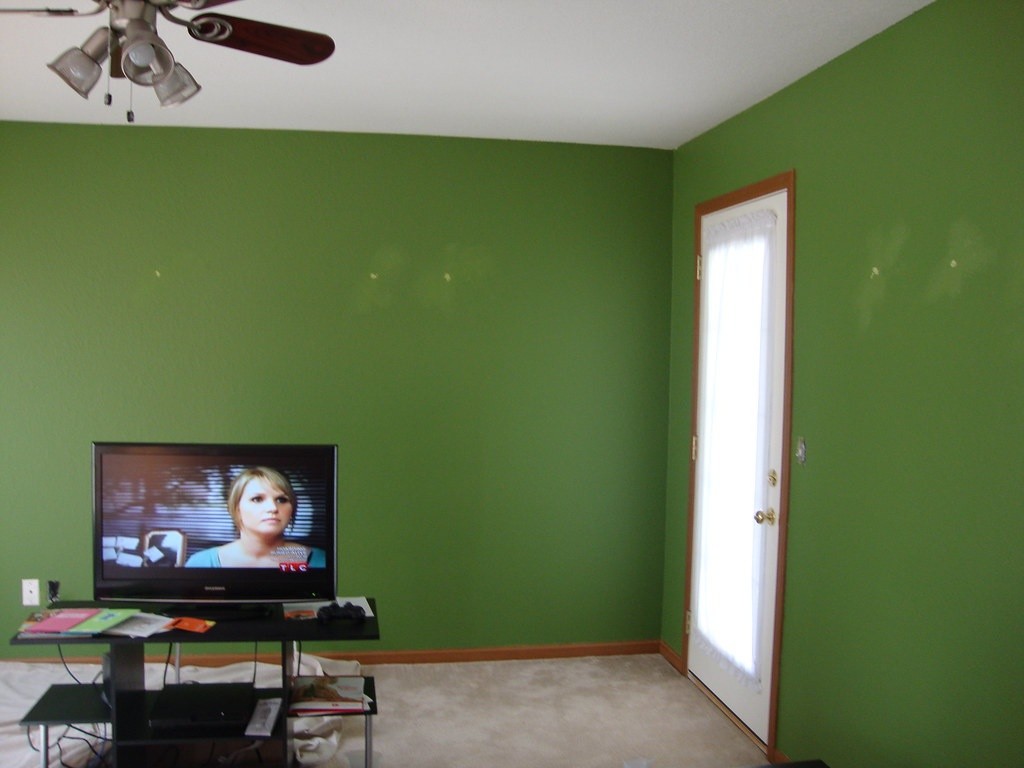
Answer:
[46,0,203,109]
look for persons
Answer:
[292,676,360,702]
[184,466,326,567]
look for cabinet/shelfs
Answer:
[9,596,380,768]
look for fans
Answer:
[0,0,336,79]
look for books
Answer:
[18,607,140,638]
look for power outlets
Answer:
[22,579,41,607]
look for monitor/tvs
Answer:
[91,441,339,621]
